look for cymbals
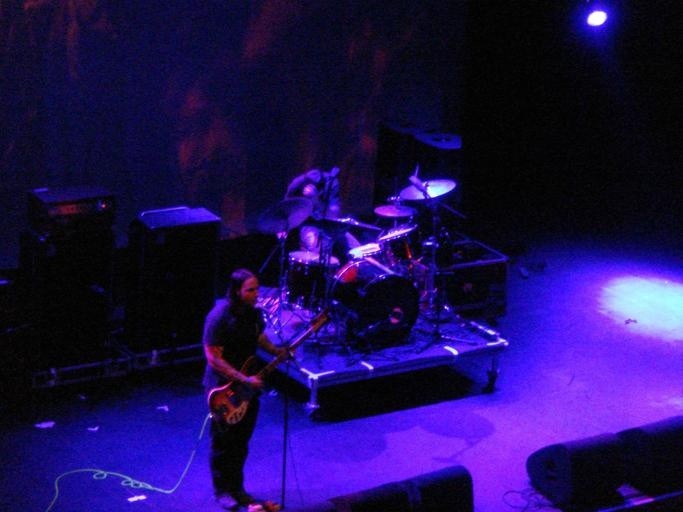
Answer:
[255,197,313,235]
[399,179,457,201]
[373,205,417,218]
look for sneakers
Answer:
[234,490,252,504]
[217,492,238,508]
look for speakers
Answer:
[525,432,630,504]
[327,464,473,512]
[619,405,682,497]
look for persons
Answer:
[285,156,361,260]
[204,267,297,511]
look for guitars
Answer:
[207,312,332,426]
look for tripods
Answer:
[264,244,477,365]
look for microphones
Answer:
[253,303,276,315]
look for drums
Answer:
[286,251,341,295]
[326,257,420,348]
[348,243,387,266]
[381,225,423,265]
